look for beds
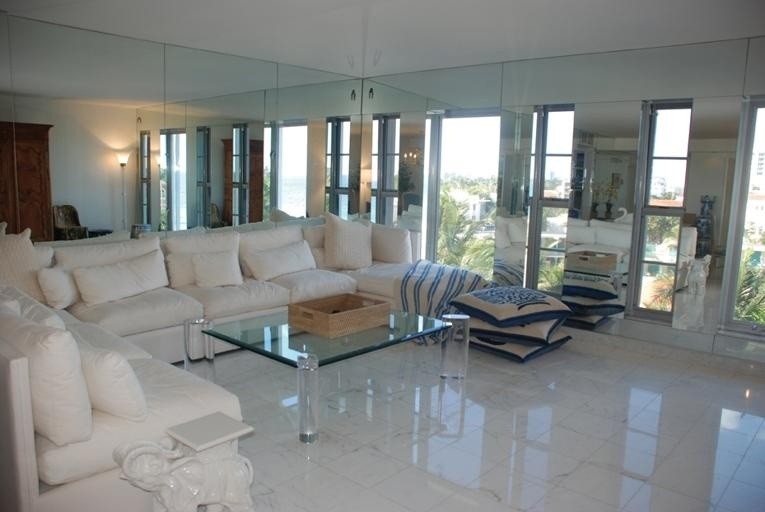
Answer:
[304,221,484,337]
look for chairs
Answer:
[52,203,114,240]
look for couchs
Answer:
[137,226,290,364]
[1,220,204,364]
[234,222,357,304]
[0,285,254,511]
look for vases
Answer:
[690,193,717,260]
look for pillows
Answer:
[165,226,317,288]
[449,287,574,365]
[38,235,169,311]
[562,273,626,330]
[323,210,413,268]
[1,280,148,446]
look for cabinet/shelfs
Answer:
[1,121,56,242]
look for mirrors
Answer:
[562,102,642,336]
[493,107,535,290]
[398,112,425,223]
[671,98,740,333]
